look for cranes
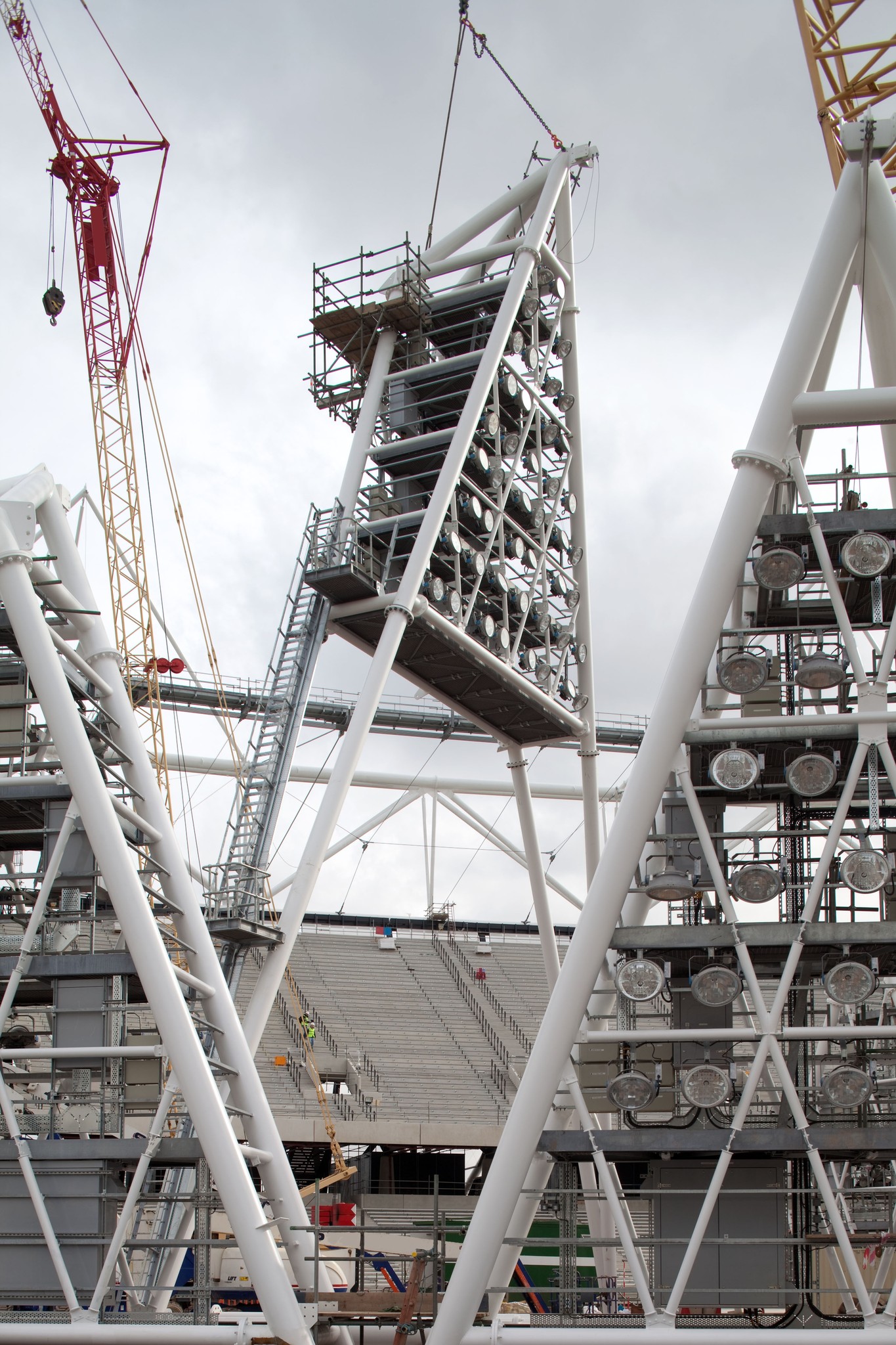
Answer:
[0,1,356,1194]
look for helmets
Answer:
[310,1022,315,1025]
[306,1011,311,1015]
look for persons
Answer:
[303,1022,316,1051]
[299,1011,311,1038]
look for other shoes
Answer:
[312,1050,314,1051]
